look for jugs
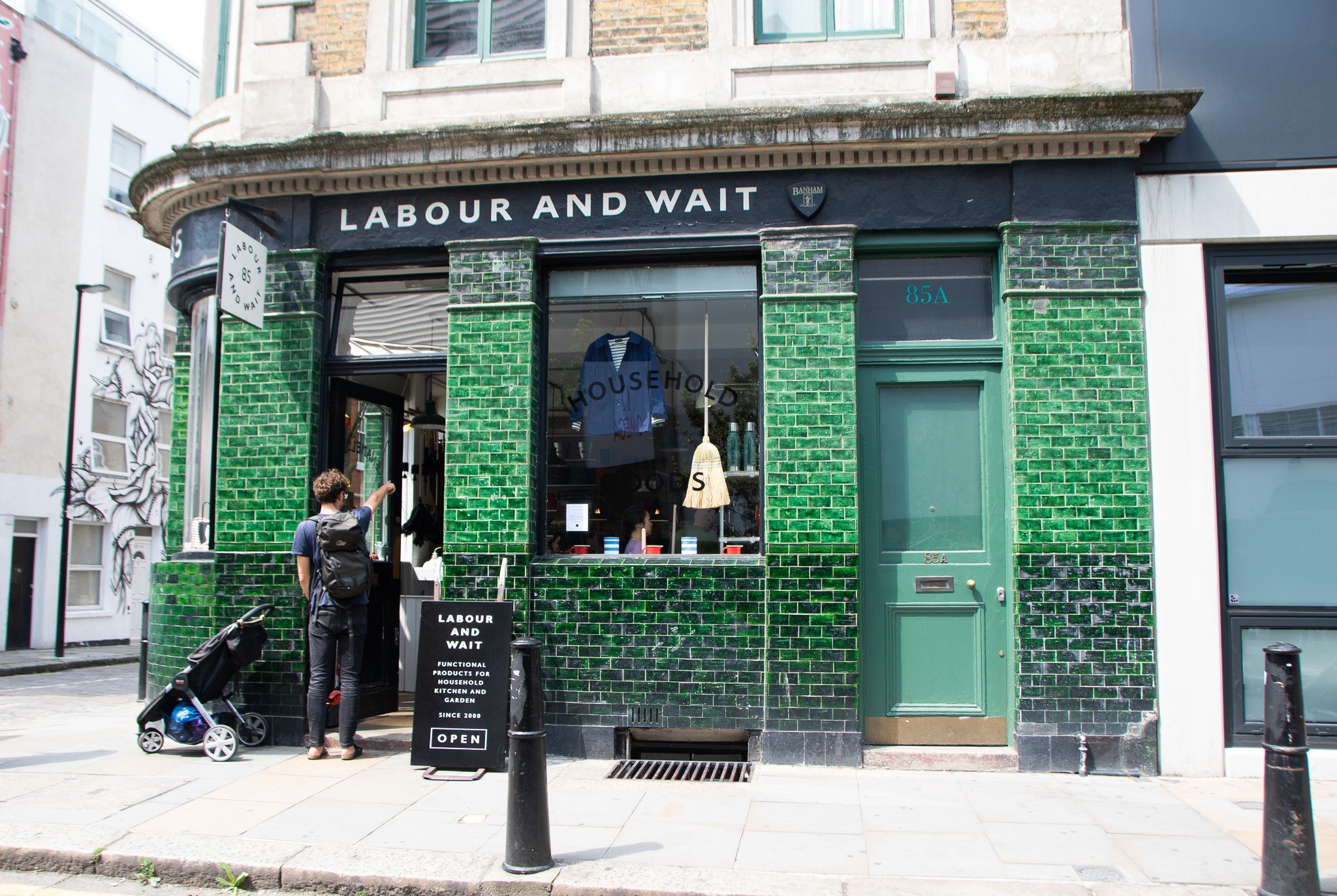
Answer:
[722,545,743,554]
[569,545,590,554]
[743,422,757,472]
[727,422,741,471]
[642,545,664,554]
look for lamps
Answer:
[404,281,447,289]
[409,372,445,431]
[695,380,719,408]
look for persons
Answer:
[551,531,587,554]
[292,470,396,760]
[622,506,652,554]
[668,509,719,554]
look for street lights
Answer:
[54,283,111,658]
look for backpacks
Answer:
[305,509,374,599]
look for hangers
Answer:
[609,303,631,343]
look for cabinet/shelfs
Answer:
[546,421,759,553]
[398,594,434,692]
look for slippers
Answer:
[306,745,326,760]
[341,743,363,759]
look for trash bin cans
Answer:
[137,600,149,702]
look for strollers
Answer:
[135,603,275,763]
